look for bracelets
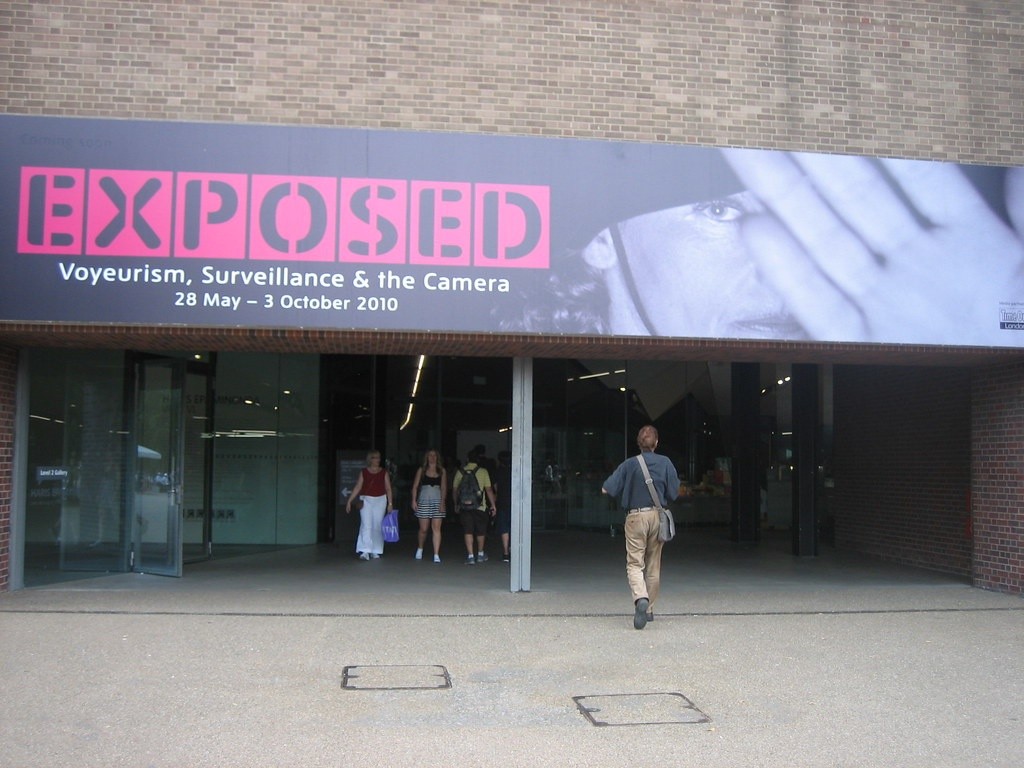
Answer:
[388,502,393,505]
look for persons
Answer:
[599,425,681,631]
[451,449,496,565]
[474,443,496,476]
[493,451,511,563]
[411,448,448,563]
[86,443,123,548]
[572,141,1024,349]
[347,450,394,560]
[134,471,170,493]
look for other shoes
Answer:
[634,599,649,630]
[477,552,488,562]
[645,613,653,621]
[464,557,475,564]
[434,554,440,562]
[370,554,379,558]
[360,553,369,560]
[415,548,423,559]
[503,555,509,562]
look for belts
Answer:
[630,506,652,513]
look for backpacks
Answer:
[459,465,483,505]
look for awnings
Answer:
[138,445,163,460]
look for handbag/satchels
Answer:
[658,509,675,542]
[350,498,363,510]
[381,510,399,542]
